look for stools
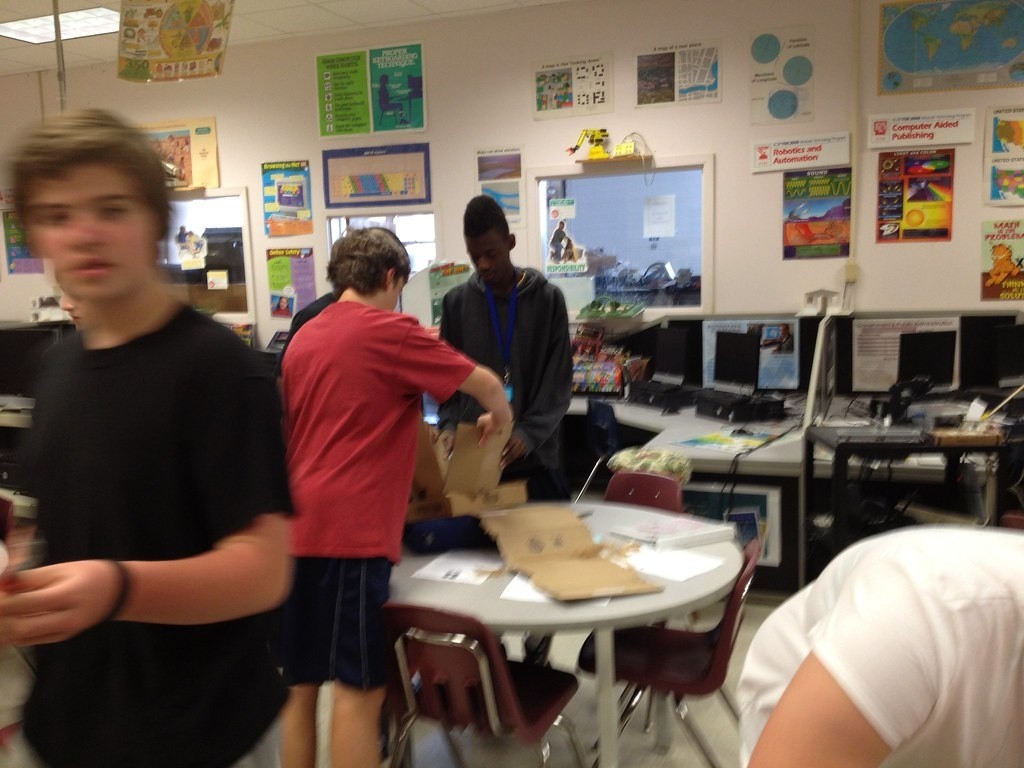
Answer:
[379,110,398,126]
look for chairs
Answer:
[529,471,688,700]
[379,599,586,768]
[578,535,768,767]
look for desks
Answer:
[567,393,1001,594]
[391,500,742,768]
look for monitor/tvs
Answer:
[651,328,690,386]
[712,332,761,397]
[899,330,958,397]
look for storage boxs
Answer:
[681,476,785,567]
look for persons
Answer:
[0,107,298,768]
[435,195,572,501]
[737,522,1024,768]
[278,227,513,768]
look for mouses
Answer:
[729,430,753,438]
[661,407,680,415]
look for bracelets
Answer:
[107,556,130,625]
